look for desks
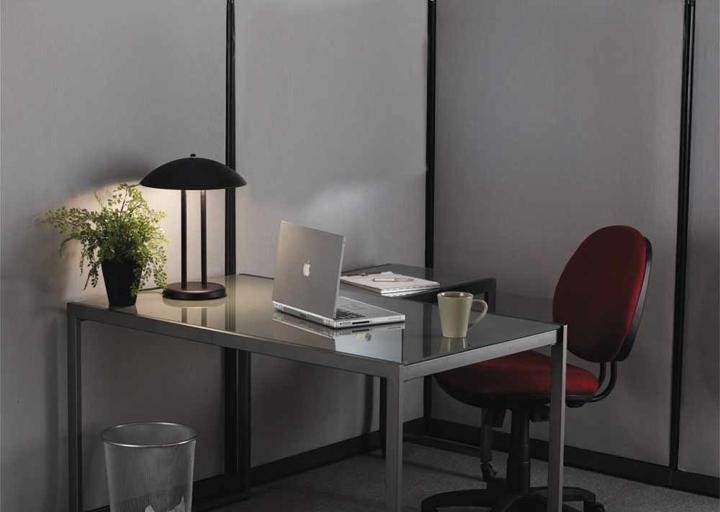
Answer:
[65,274,570,512]
[241,262,497,499]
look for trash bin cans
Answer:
[101,420,201,512]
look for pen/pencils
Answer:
[347,271,382,275]
[373,278,414,282]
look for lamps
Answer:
[139,153,248,300]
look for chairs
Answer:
[421,224,652,512]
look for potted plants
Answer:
[28,180,172,307]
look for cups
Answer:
[438,291,489,339]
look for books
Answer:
[340,271,441,294]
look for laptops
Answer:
[271,221,406,330]
